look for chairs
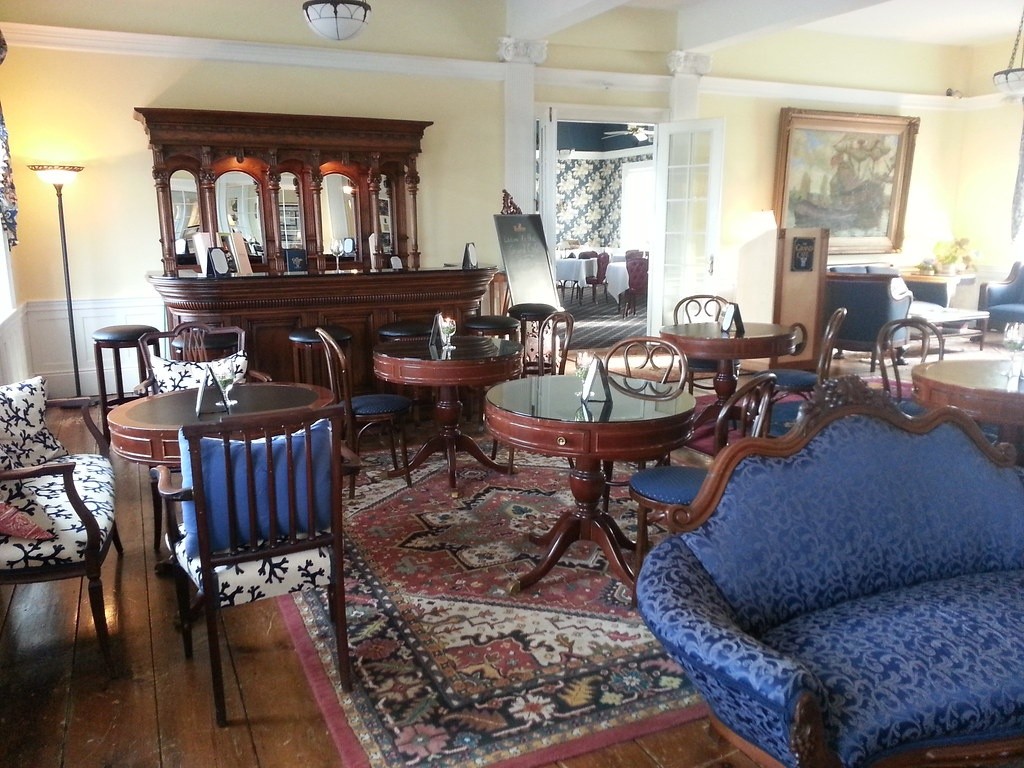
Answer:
[148,406,350,726]
[135,321,274,548]
[491,247,944,609]
[0,397,123,665]
[313,327,417,500]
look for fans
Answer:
[602,123,656,142]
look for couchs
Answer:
[635,375,1024,768]
[831,264,951,337]
[970,263,1024,343]
[827,272,914,367]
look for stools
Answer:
[91,302,560,446]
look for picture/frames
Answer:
[770,107,921,256]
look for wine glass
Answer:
[330,239,344,273]
[212,359,238,405]
[442,319,456,350]
[574,351,595,397]
[1002,323,1024,378]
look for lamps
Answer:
[28,164,100,408]
[303,0,371,43]
[994,9,1024,95]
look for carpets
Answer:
[279,383,1024,768]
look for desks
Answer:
[372,335,524,500]
[910,360,1024,449]
[107,384,336,627]
[659,322,799,434]
[556,259,598,307]
[605,261,630,313]
[485,374,697,597]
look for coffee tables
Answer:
[912,309,990,351]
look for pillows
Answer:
[179,419,333,559]
[1,449,57,540]
[0,375,67,467]
[150,350,249,394]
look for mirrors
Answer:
[134,107,423,271]
